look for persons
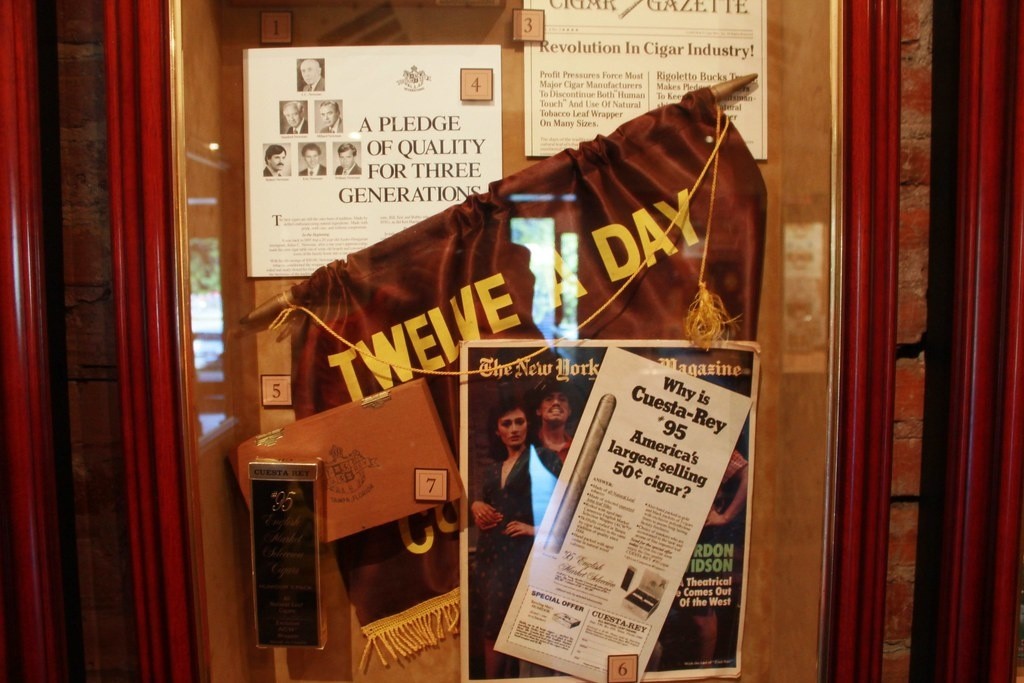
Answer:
[263,145,287,177]
[648,446,749,673]
[319,100,343,133]
[300,59,325,92]
[335,143,362,176]
[469,381,577,680]
[298,143,326,176]
[282,101,308,134]
